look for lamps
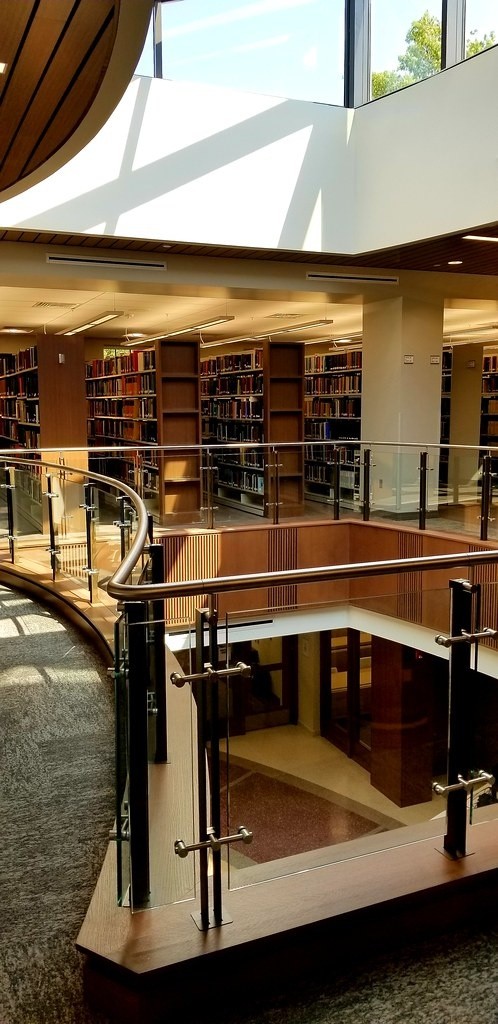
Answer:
[58,309,122,336]
[123,315,234,347]
[199,319,334,348]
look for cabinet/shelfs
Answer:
[0,333,498,534]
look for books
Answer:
[85,350,159,502]
[200,350,263,493]
[0,345,42,504]
[481,356,498,470]
[304,351,362,489]
[440,351,452,461]
